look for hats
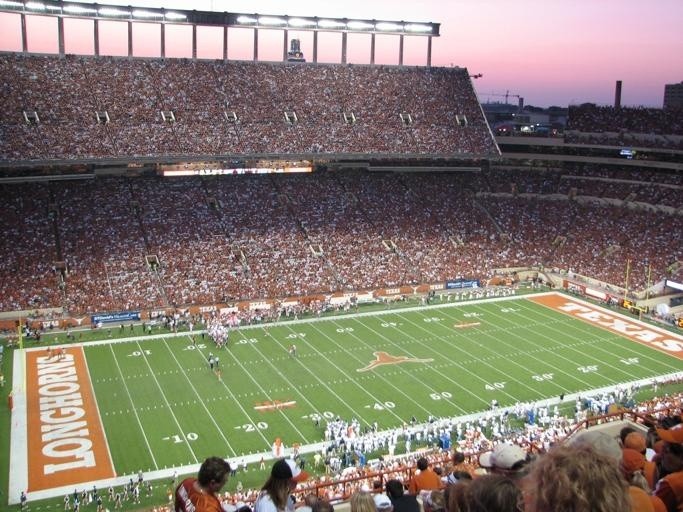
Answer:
[442,472,458,485]
[477,443,528,470]
[619,424,682,475]
[269,459,308,484]
[372,494,392,509]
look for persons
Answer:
[0,50,683,512]
